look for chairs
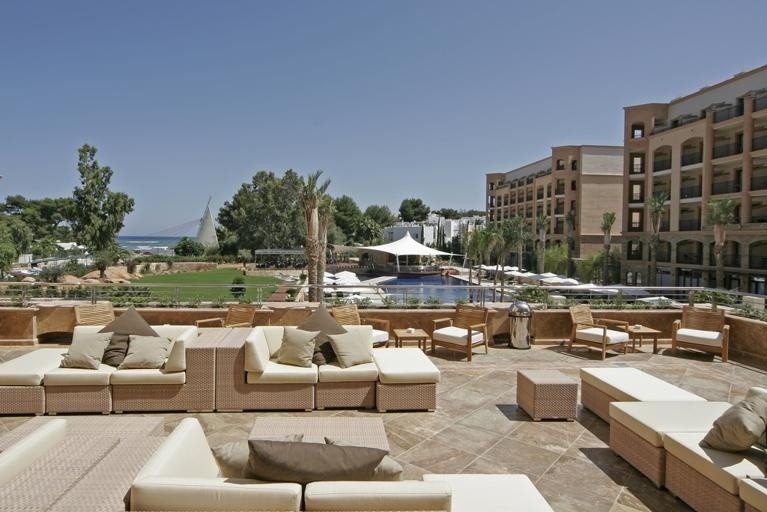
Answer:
[431,304,488,361]
[567,305,630,359]
[671,305,730,362]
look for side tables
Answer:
[616,325,662,354]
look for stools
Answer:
[516,369,579,422]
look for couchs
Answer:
[580,367,767,512]
[0,305,440,416]
[130,416,555,512]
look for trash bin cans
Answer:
[508,302,533,349]
